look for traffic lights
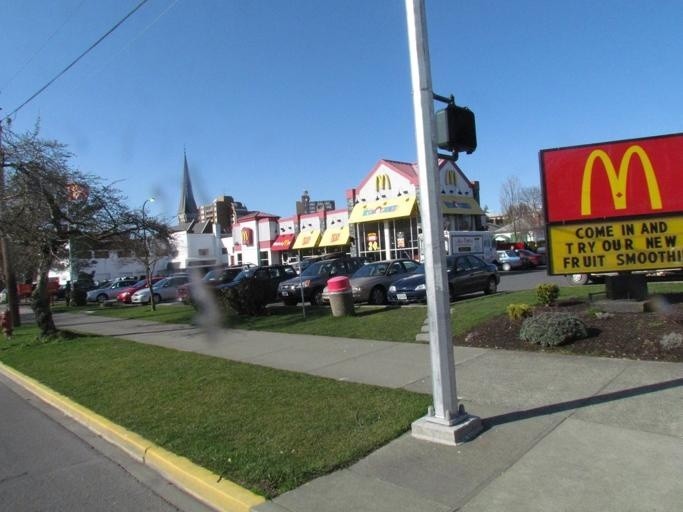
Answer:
[437,105,478,153]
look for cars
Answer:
[518,249,544,270]
[132,275,192,305]
[388,253,501,305]
[52,280,94,300]
[569,268,681,286]
[117,277,165,304]
[322,259,420,305]
[284,252,348,275]
[495,250,522,271]
[86,277,137,303]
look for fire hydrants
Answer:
[0,307,15,338]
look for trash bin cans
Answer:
[327,276,355,317]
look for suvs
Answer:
[176,267,242,305]
[278,258,369,306]
[193,265,298,313]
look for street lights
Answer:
[142,196,157,274]
